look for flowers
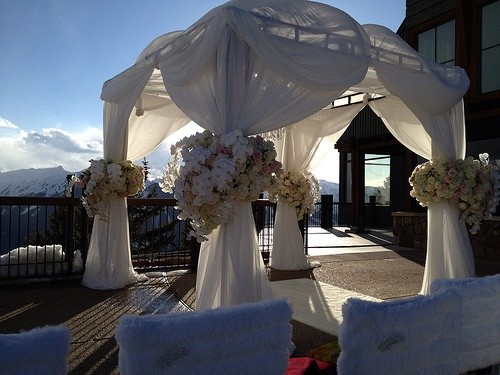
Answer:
[409,155,497,235]
[157,128,314,242]
[65,157,144,224]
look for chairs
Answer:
[116,299,293,375]
[0,325,73,375]
[331,273,500,375]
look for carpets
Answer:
[268,278,387,336]
